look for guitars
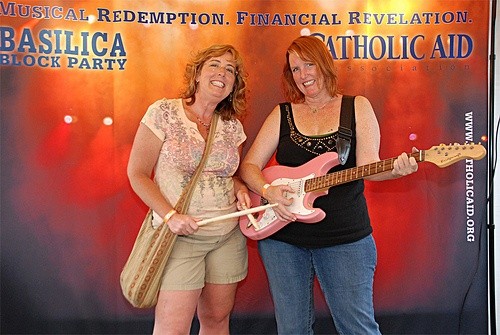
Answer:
[239,142,487,241]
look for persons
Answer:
[241,36,419,335]
[127,44,253,335]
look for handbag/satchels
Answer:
[118,208,190,310]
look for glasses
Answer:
[199,62,238,76]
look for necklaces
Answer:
[184,106,213,127]
[304,98,334,113]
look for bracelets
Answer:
[261,183,270,197]
[163,209,176,224]
